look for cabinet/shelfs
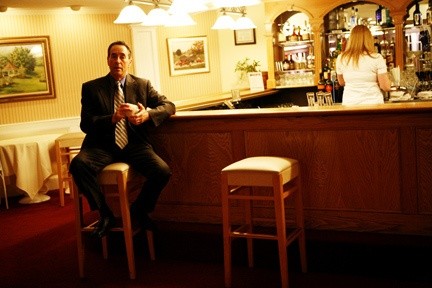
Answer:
[276,22,432,72]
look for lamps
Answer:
[209,7,257,30]
[112,0,195,28]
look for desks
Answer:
[0,133,65,205]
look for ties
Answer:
[114,80,128,149]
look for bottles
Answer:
[282,24,315,70]
[317,5,396,92]
[413,0,431,26]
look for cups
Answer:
[230,88,241,101]
[306,91,333,106]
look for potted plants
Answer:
[234,58,268,88]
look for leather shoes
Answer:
[96,213,116,236]
[131,208,157,229]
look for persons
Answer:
[335,25,392,105]
[68,41,177,239]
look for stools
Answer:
[72,162,154,282]
[220,156,305,288]
[55,133,86,206]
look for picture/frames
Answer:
[0,36,57,104]
[167,36,210,77]
[234,28,257,46]
